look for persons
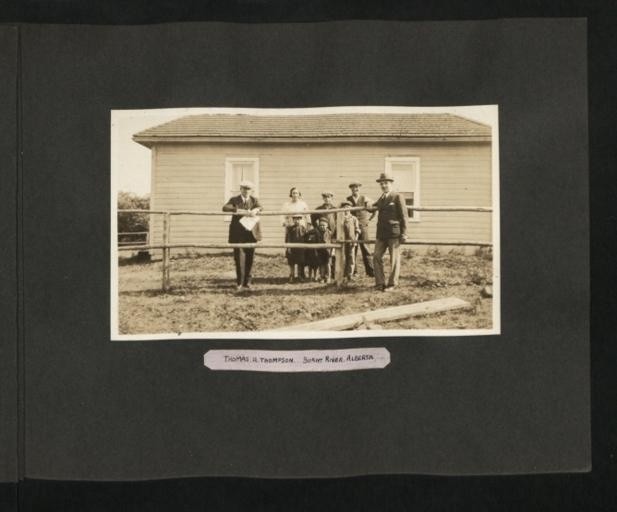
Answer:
[221,181,263,293]
[279,182,375,284]
[363,173,409,292]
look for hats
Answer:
[376,172,394,182]
[321,191,333,198]
[319,216,329,223]
[349,182,362,188]
[239,183,253,189]
[340,201,352,207]
[292,214,303,219]
